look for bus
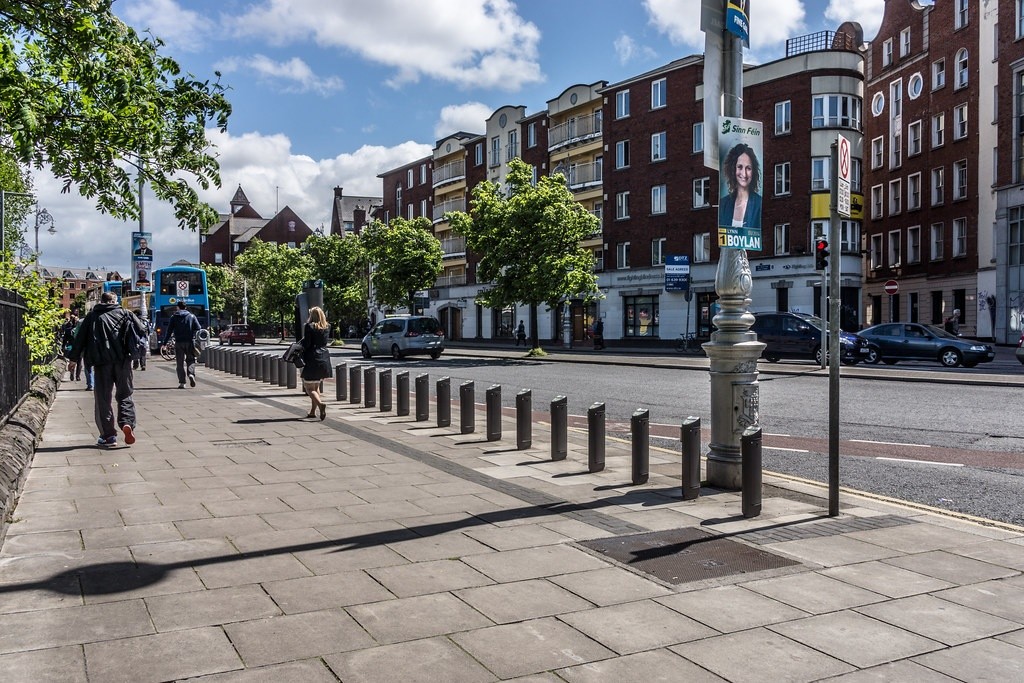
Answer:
[84,280,122,317]
[122,265,212,349]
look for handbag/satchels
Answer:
[121,308,147,359]
[283,337,306,368]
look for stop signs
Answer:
[884,279,899,296]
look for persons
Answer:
[787,320,802,332]
[133,309,152,371]
[719,143,762,228]
[515,320,527,347]
[67,291,143,448]
[161,301,201,389]
[135,270,150,286]
[591,317,607,350]
[61,314,95,391]
[299,306,333,422]
[134,238,152,255]
[945,309,961,336]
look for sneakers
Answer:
[122,425,137,446]
[97,436,118,447]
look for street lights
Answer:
[34,206,58,270]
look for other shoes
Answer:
[141,366,146,371]
[133,366,138,370]
[178,383,186,388]
[320,404,326,421]
[86,387,94,391]
[189,374,196,387]
[76,378,81,381]
[308,412,317,417]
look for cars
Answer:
[853,322,996,368]
[1015,334,1024,364]
[265,326,286,338]
[218,323,256,346]
[750,309,871,366]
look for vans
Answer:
[361,315,446,360]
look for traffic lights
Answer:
[815,236,829,272]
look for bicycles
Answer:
[674,331,702,352]
[160,333,202,362]
